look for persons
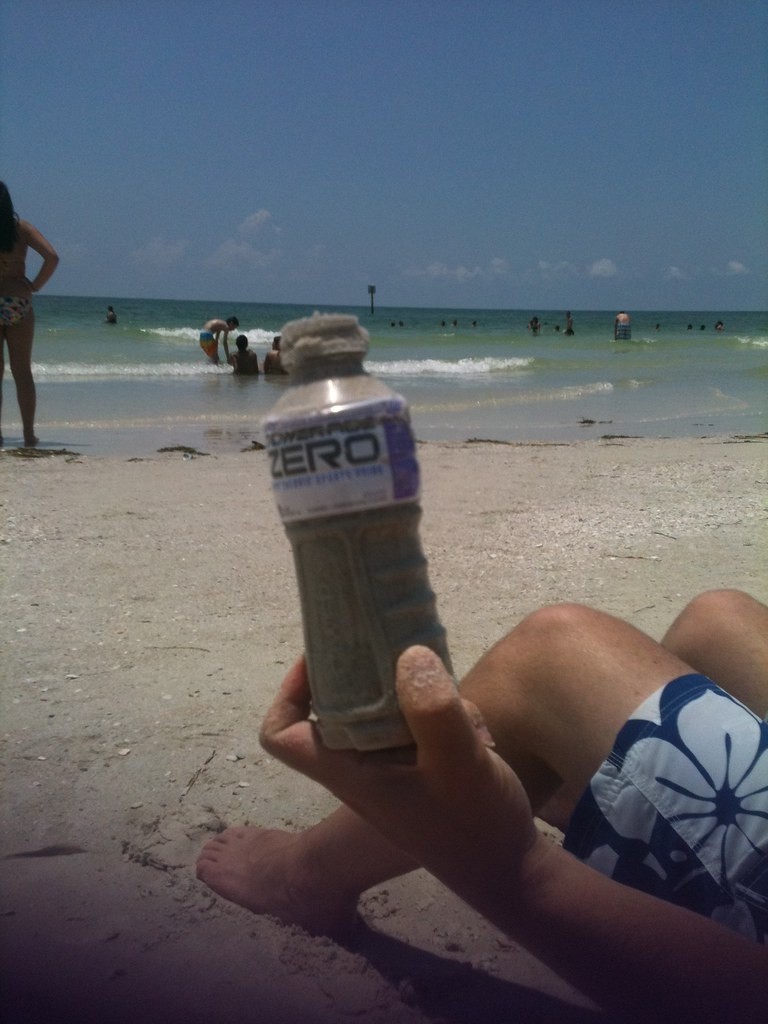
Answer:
[528,316,540,335]
[199,315,239,366]
[196,586,767,1023]
[715,321,725,332]
[564,311,574,335]
[0,176,61,451]
[263,334,290,376]
[230,335,260,375]
[104,305,116,322]
[613,310,632,340]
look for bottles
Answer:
[263,315,455,751]
